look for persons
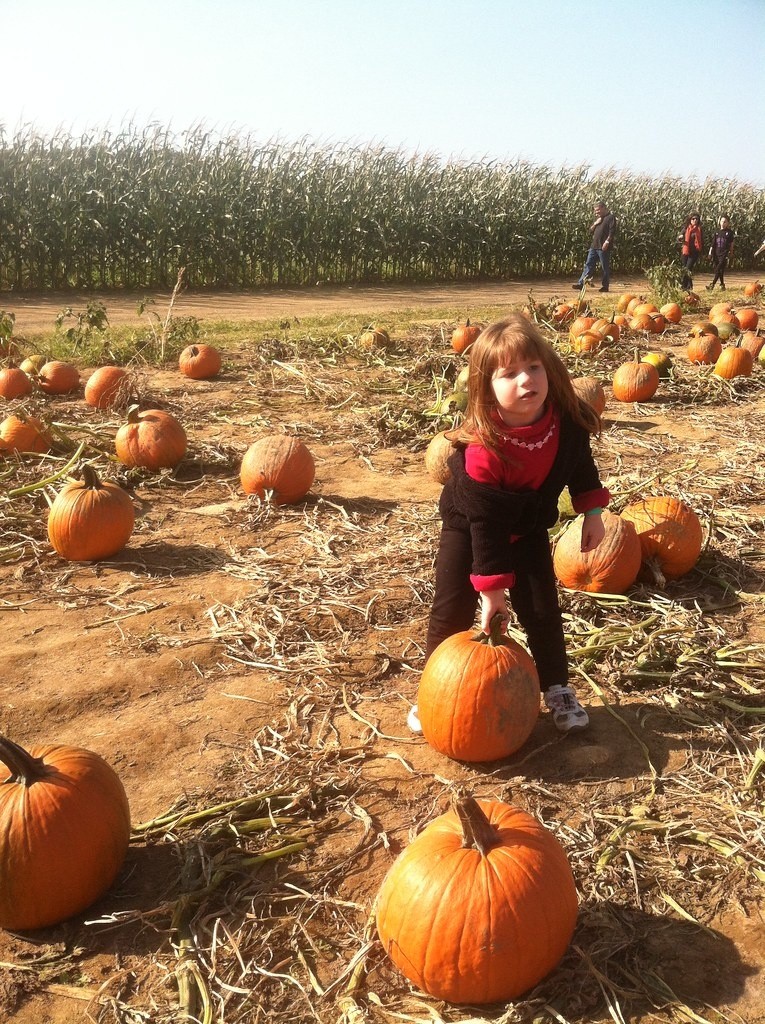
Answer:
[408,315,611,733]
[676,212,705,290]
[572,201,617,292]
[754,239,765,258]
[706,215,734,292]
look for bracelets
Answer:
[582,507,602,515]
[593,222,597,226]
[605,240,610,244]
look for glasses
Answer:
[690,218,698,220]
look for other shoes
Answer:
[599,287,608,292]
[572,284,582,290]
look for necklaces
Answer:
[493,415,556,451]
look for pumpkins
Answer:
[360,327,389,348]
[615,496,703,589]
[417,612,541,761]
[373,783,578,1005]
[0,343,314,561]
[425,416,462,485]
[447,279,765,415]
[554,510,641,594]
[0,731,130,930]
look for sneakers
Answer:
[717,284,726,292]
[407,704,424,736]
[543,684,591,735]
[705,283,714,290]
